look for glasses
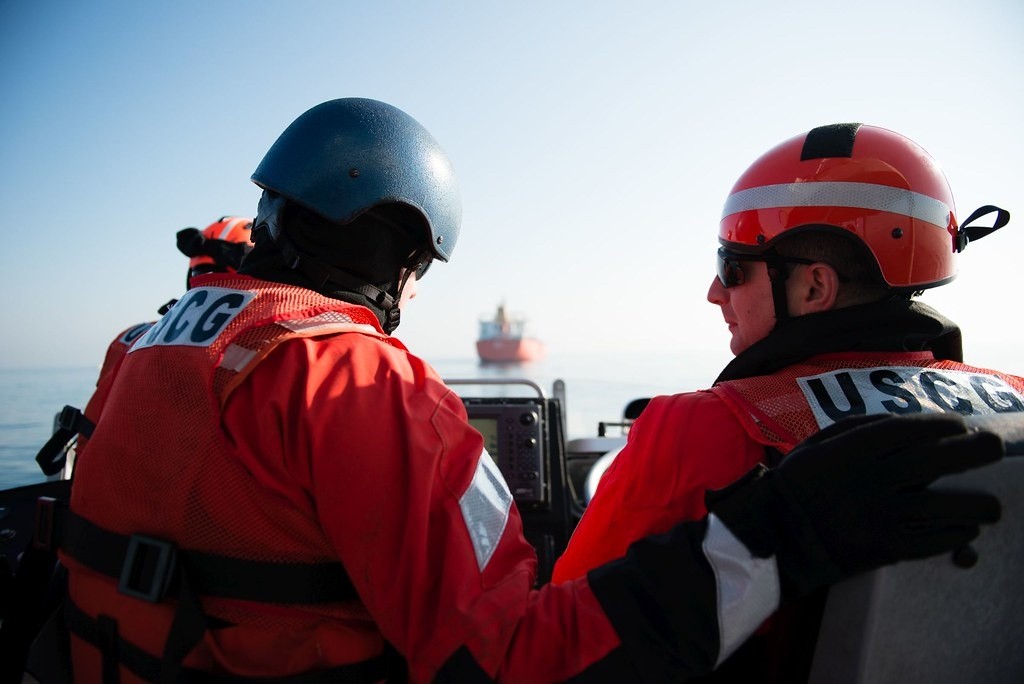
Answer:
[717,248,849,290]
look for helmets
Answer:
[249,97,462,263]
[717,123,952,294]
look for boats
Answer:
[475,302,544,363]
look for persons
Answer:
[549,122,1024,684]
[71,214,263,473]
[56,96,1008,684]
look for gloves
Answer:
[704,412,1006,586]
